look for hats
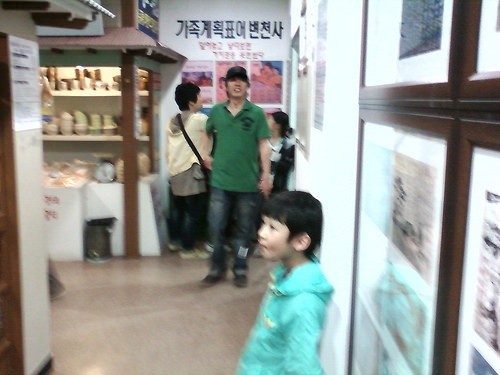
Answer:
[224,66,248,82]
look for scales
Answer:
[94,153,116,183]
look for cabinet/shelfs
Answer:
[41,88,149,143]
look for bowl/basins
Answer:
[113,75,149,91]
[62,79,77,89]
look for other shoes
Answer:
[235,274,248,286]
[201,274,220,285]
[180,247,210,259]
[167,240,183,251]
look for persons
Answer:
[164,81,296,261]
[234,191,334,375]
[199,65,273,288]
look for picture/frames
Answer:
[347,0,500,375]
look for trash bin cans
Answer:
[85,224,112,264]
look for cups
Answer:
[108,82,119,91]
[95,81,107,91]
[70,80,80,91]
[58,82,67,91]
[42,111,118,136]
[82,78,93,90]
